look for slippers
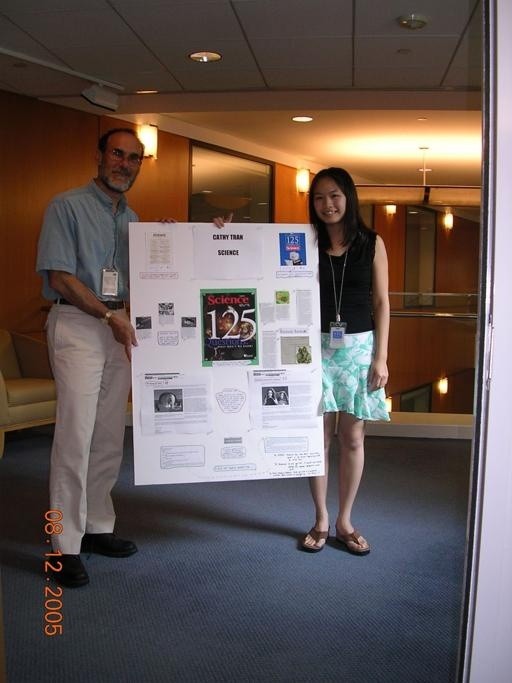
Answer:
[336,528,370,555]
[302,525,330,551]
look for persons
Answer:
[276,390,289,405]
[263,388,279,405]
[33,124,179,591]
[209,164,394,556]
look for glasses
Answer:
[108,149,140,166]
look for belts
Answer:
[54,297,125,309]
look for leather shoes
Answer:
[81,532,138,556]
[48,551,89,587]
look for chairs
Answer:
[0,328,59,460]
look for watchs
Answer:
[104,308,114,321]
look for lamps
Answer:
[445,214,453,231]
[296,168,310,192]
[79,83,118,112]
[138,124,157,158]
[386,205,396,214]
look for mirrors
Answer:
[354,183,481,319]
[187,136,277,223]
